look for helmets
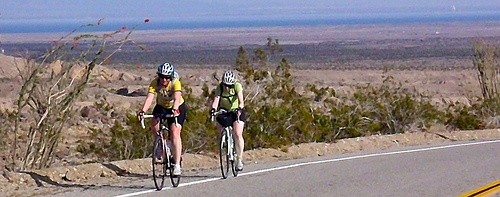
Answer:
[222,71,236,85]
[157,62,174,77]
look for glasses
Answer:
[158,73,171,79]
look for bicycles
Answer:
[137,110,183,191]
[209,108,241,179]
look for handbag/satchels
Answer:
[217,110,237,126]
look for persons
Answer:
[138,62,189,176]
[209,70,246,171]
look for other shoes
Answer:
[155,155,162,164]
[235,160,244,171]
[170,162,182,176]
